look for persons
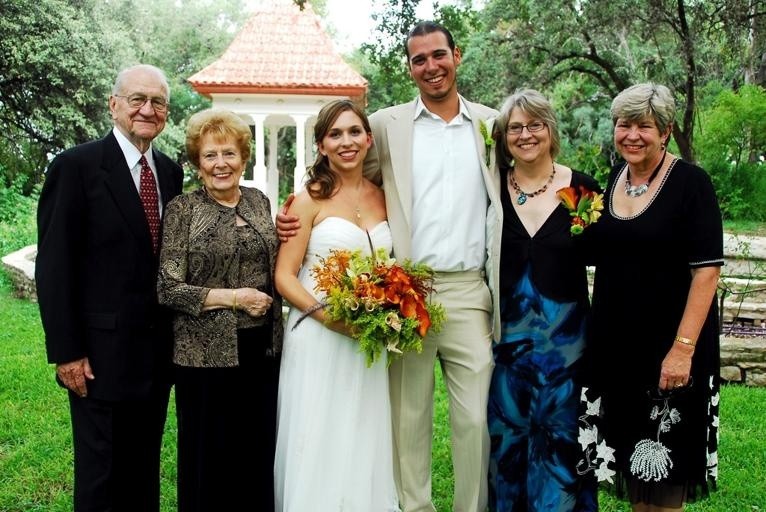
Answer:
[272,98,400,512]
[31,63,187,511]
[157,108,285,512]
[488,88,605,511]
[274,19,503,512]
[575,79,724,512]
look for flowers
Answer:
[557,184,605,235]
[290,225,449,374]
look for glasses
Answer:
[506,118,548,134]
[115,92,170,110]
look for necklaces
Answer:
[335,176,366,220]
[508,157,557,206]
[623,149,667,198]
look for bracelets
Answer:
[675,334,698,348]
[229,288,238,314]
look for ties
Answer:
[138,156,160,257]
